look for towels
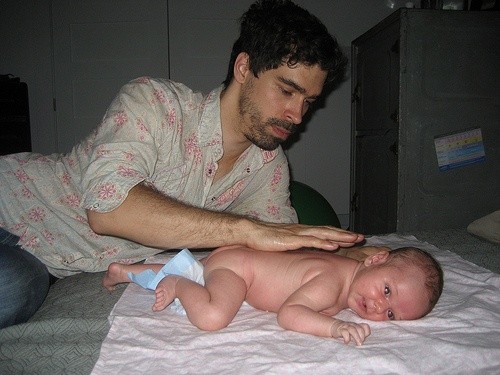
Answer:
[90,233,500,375]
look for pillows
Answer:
[467,210,500,244]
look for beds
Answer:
[0,229,500,375]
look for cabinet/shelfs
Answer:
[348,9,500,232]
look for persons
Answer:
[0,0,365,328]
[103,245,443,346]
